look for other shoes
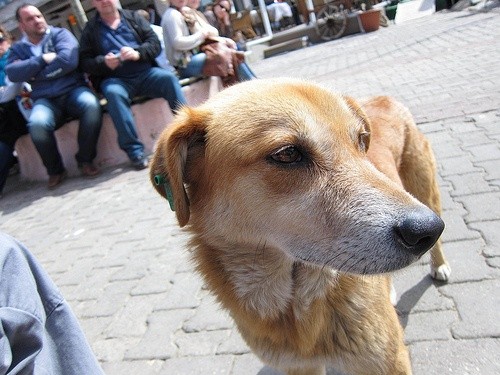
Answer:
[134,160,149,171]
[45,170,69,191]
[77,161,103,179]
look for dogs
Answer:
[148,76,452,375]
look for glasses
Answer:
[217,3,229,13]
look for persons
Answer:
[0,231,106,375]
[0,0,257,193]
